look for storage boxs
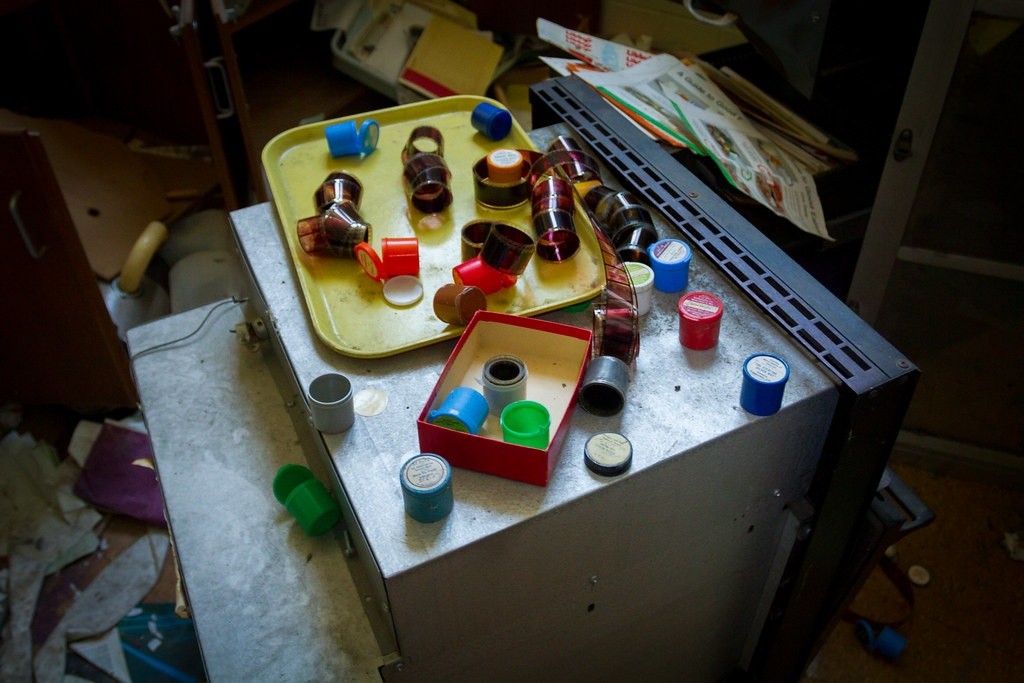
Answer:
[417,311,592,488]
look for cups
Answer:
[648,239,694,294]
[616,263,655,315]
[306,373,355,434]
[501,401,549,450]
[485,355,530,415]
[432,387,491,435]
[680,293,725,350]
[741,353,790,417]
[580,356,630,417]
[434,285,486,328]
[272,463,338,535]
[354,238,420,278]
[487,149,522,183]
[453,256,519,295]
[854,620,906,661]
[400,453,455,522]
[471,104,512,143]
[325,121,379,157]
